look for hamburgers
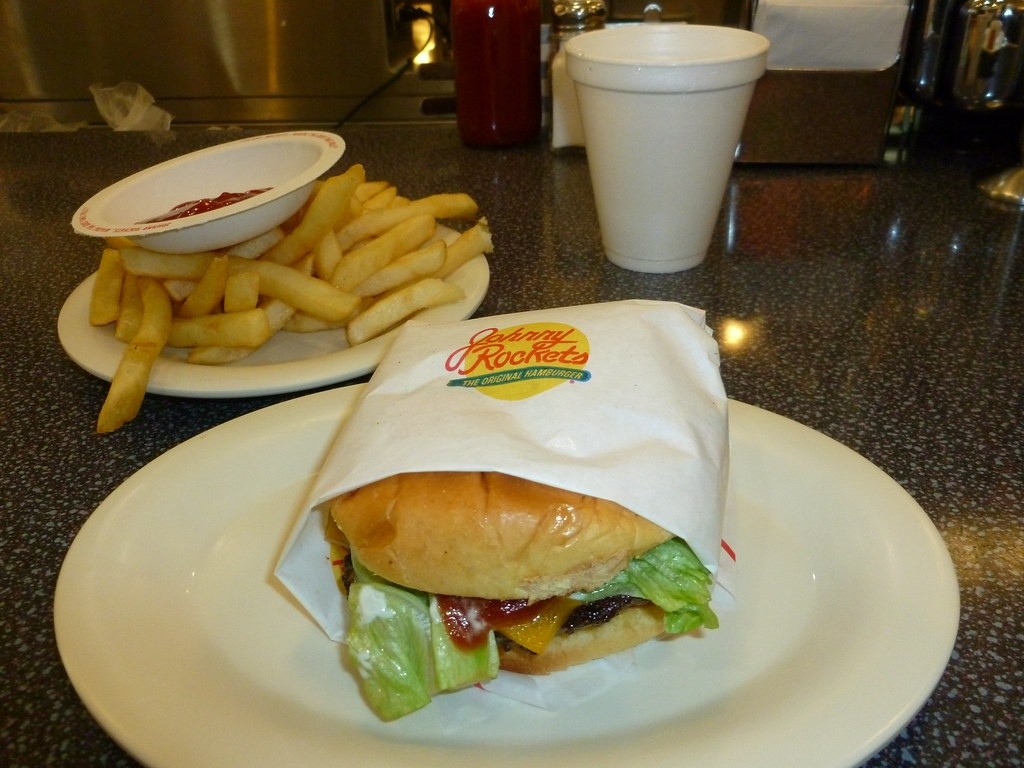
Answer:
[323,472,718,721]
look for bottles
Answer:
[549,0,608,155]
[896,0,1024,110]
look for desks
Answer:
[1,120,1024,768]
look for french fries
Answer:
[90,163,494,433]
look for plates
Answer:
[53,383,960,768]
[71,130,347,253]
[56,222,489,396]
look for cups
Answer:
[567,24,768,273]
[449,0,541,147]
